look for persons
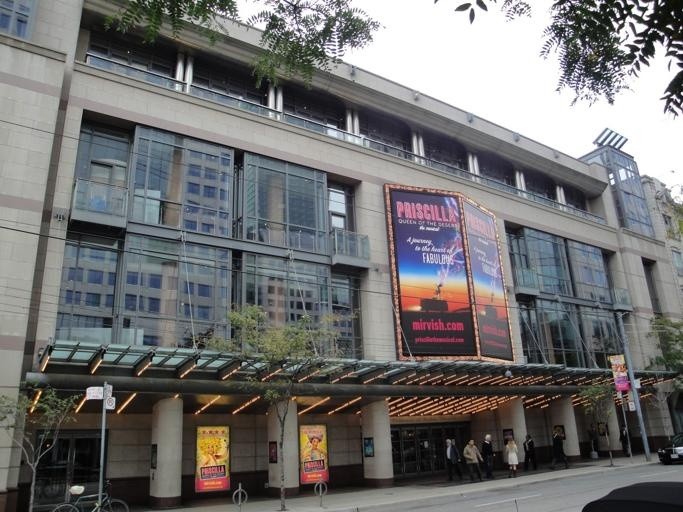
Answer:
[481,433,496,479]
[619,423,629,457]
[505,438,518,477]
[523,434,537,472]
[462,439,484,481]
[445,438,460,481]
[550,427,569,470]
[303,433,326,461]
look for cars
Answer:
[578,479,683,512]
[655,431,682,465]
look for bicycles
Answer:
[50,478,130,512]
